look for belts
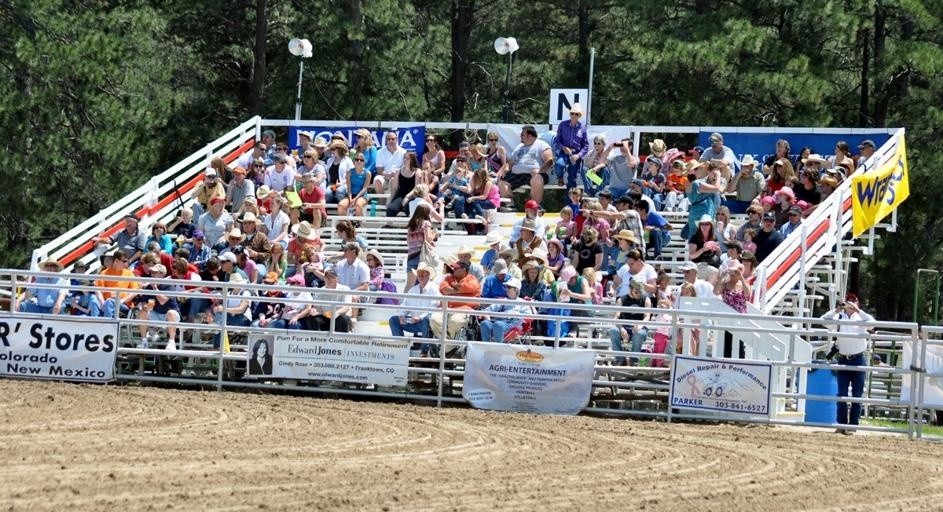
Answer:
[837,350,865,362]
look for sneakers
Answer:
[165,339,176,352]
[135,337,149,349]
[833,426,845,433]
[843,427,857,435]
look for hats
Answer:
[409,261,437,281]
[203,129,380,291]
[454,133,847,309]
[36,259,64,273]
[856,140,875,153]
[71,213,202,283]
[566,102,586,119]
[844,295,859,307]
[873,354,880,360]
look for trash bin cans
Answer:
[805,359,840,424]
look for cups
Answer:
[148,298,154,308]
[559,225,568,237]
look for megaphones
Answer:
[288,38,313,58]
[493,36,518,56]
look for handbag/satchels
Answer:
[416,240,439,268]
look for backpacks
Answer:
[374,278,400,307]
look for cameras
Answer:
[730,269,737,275]
[826,345,839,360]
[835,300,846,308]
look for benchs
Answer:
[315,169,896,397]
[116,324,248,386]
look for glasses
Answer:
[425,139,433,143]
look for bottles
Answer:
[459,328,465,340]
[370,201,377,218]
